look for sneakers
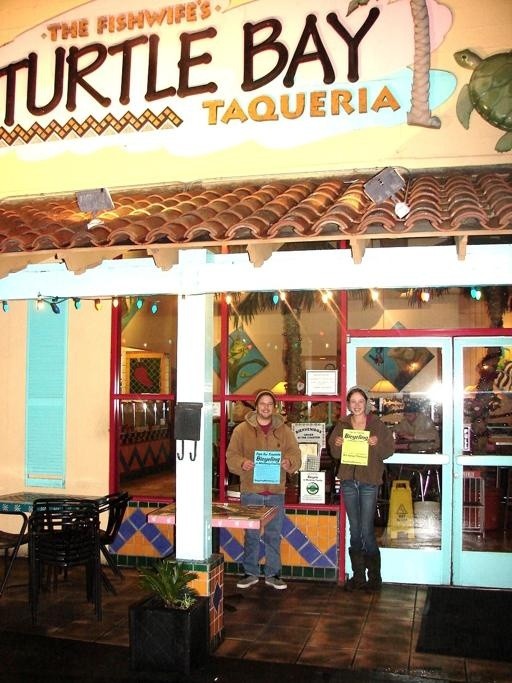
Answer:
[236,573,260,588]
[264,576,288,589]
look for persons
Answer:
[225,389,301,590]
[328,388,393,592]
[388,402,441,475]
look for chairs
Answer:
[401,441,441,503]
[1,492,132,621]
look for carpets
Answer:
[416,588,510,661]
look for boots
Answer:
[344,548,367,592]
[365,550,382,592]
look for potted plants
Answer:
[128,558,211,681]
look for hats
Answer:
[403,401,420,412]
[254,389,276,407]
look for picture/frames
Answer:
[298,440,327,505]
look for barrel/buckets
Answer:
[412,500,441,541]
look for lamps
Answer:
[368,289,398,393]
[361,163,411,219]
[74,188,115,229]
